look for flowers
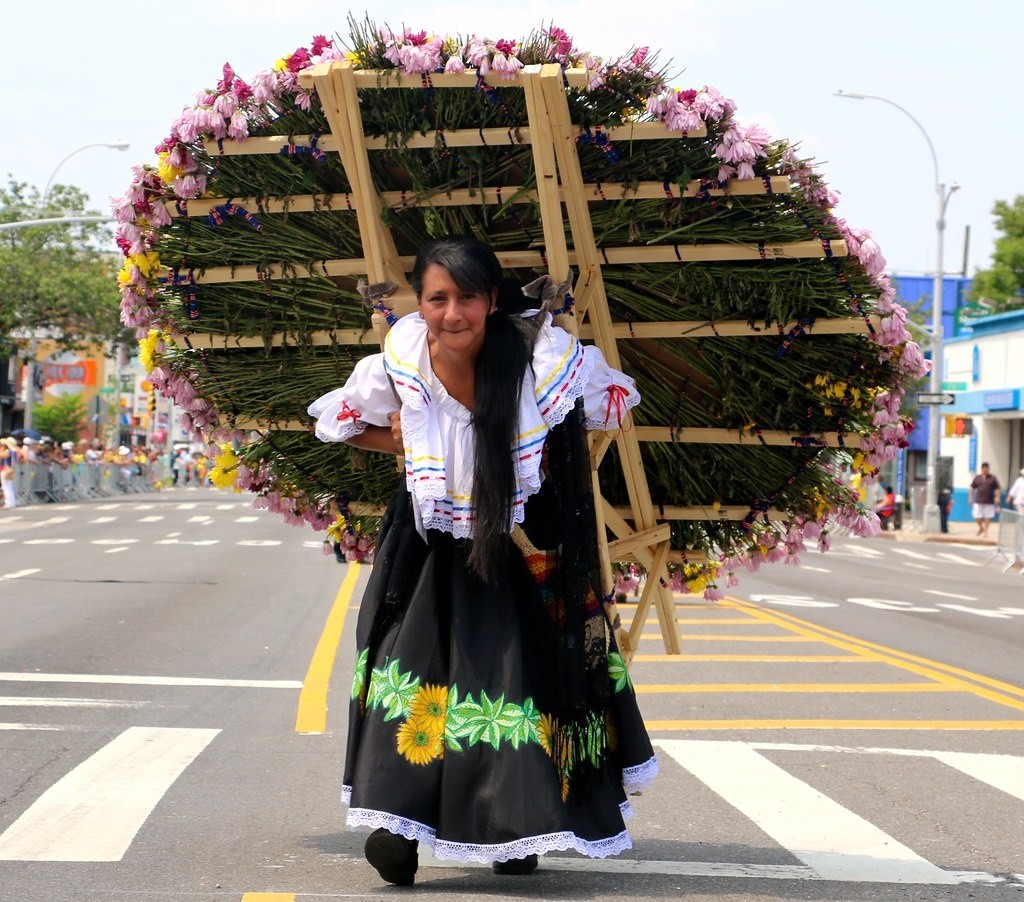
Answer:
[101,5,938,599]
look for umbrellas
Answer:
[12,429,41,441]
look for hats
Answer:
[0,435,131,455]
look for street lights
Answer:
[40,141,130,215]
[835,92,965,535]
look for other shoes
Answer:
[365,829,418,886]
[492,849,538,873]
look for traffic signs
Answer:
[916,392,956,405]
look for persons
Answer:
[866,475,894,530]
[969,463,1001,534]
[0,430,214,506]
[1005,467,1024,513]
[309,237,656,885]
